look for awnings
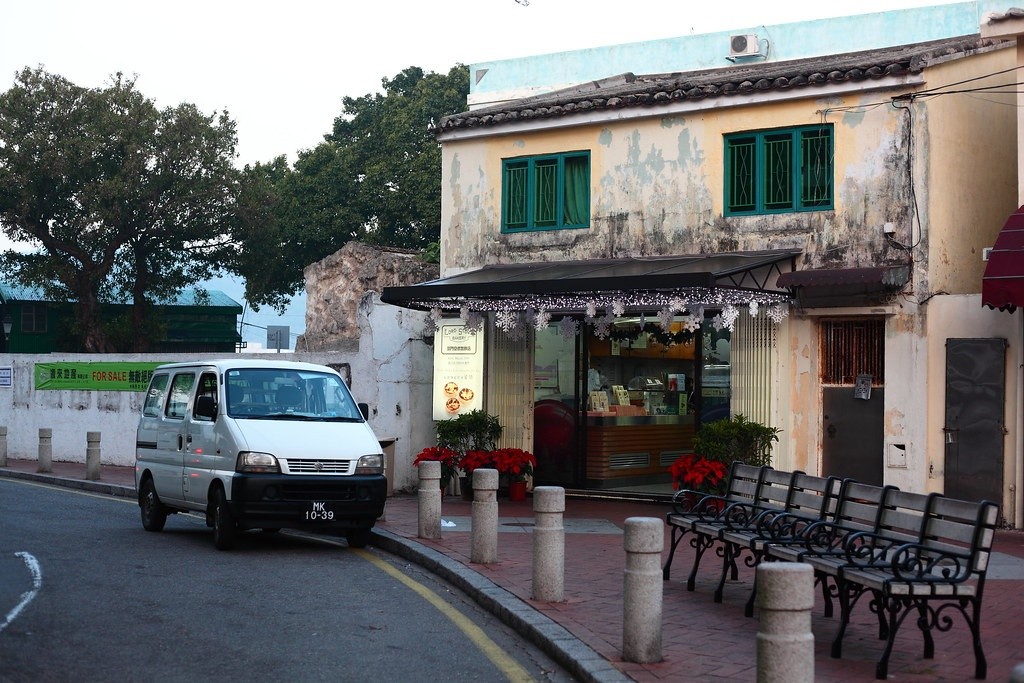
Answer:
[380,248,809,310]
[980,205,1024,314]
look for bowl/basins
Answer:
[458,388,474,404]
[445,397,461,414]
[443,382,458,398]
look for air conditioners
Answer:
[729,34,759,59]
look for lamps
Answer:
[0,314,14,337]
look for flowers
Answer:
[412,447,460,488]
[458,450,493,473]
[665,451,695,492]
[684,456,728,498]
[493,448,536,481]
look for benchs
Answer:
[763,477,1000,681]
[663,461,842,618]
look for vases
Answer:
[439,488,445,502]
[682,493,702,512]
[508,481,527,501]
[705,496,725,513]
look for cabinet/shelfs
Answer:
[590,322,705,359]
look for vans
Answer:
[134,357,389,552]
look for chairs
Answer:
[275,386,302,413]
[215,384,249,410]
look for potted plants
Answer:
[434,409,507,500]
